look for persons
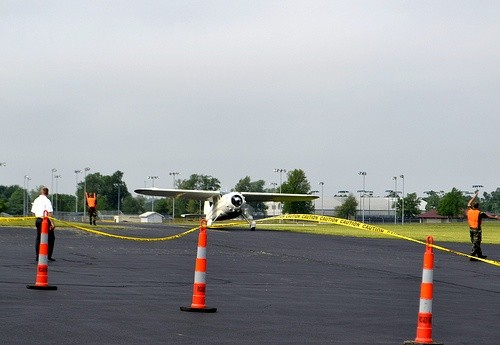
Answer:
[85,192,97,226]
[467,193,500,261]
[31,188,56,262]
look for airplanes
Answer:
[134,188,320,231]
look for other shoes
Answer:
[470,258,478,262]
[478,255,487,259]
[48,258,56,263]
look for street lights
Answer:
[318,181,324,216]
[53,174,62,211]
[146,175,160,211]
[112,181,125,215]
[400,174,405,224]
[0,162,7,168]
[50,168,57,205]
[392,176,398,224]
[85,166,91,219]
[72,169,83,212]
[23,175,32,217]
[168,171,184,219]
[273,168,286,215]
[358,171,367,223]
